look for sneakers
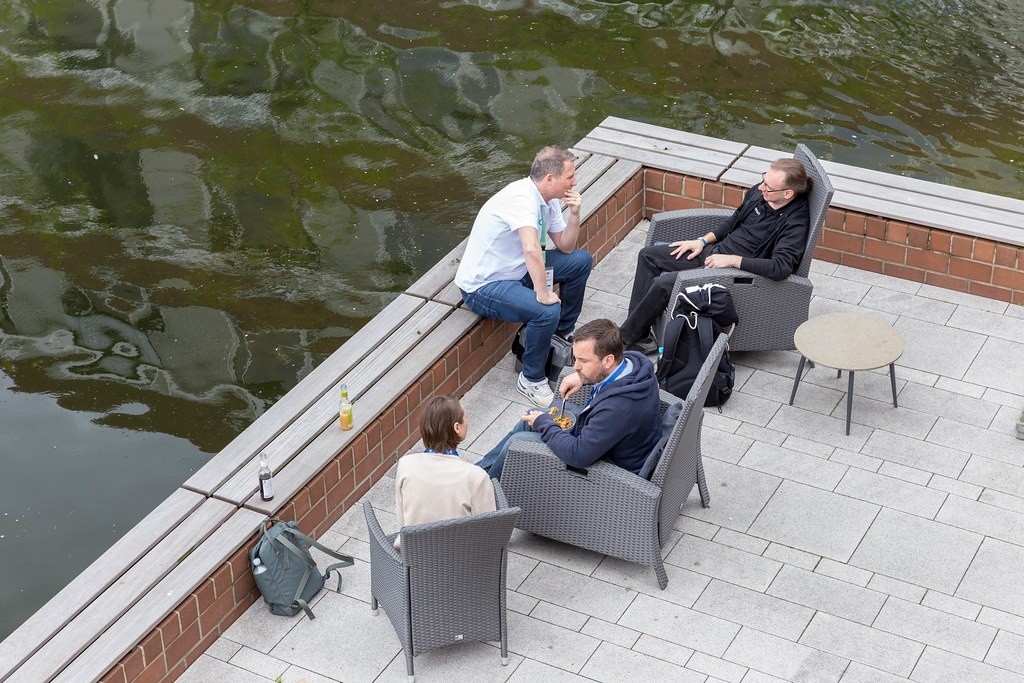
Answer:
[516,372,554,408]
[563,329,576,340]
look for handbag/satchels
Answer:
[515,326,576,382]
[670,283,739,327]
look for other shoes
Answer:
[624,332,658,354]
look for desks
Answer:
[789,313,904,436]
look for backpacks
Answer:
[249,519,354,620]
[654,316,735,414]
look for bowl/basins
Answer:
[544,409,576,434]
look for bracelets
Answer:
[697,238,706,246]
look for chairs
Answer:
[362,479,522,683]
[497,321,739,589]
[644,144,837,353]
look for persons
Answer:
[619,158,813,355]
[454,146,593,408]
[392,395,497,551]
[474,318,662,485]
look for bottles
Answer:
[259,453,275,501]
[338,384,354,430]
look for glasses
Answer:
[762,172,796,194]
